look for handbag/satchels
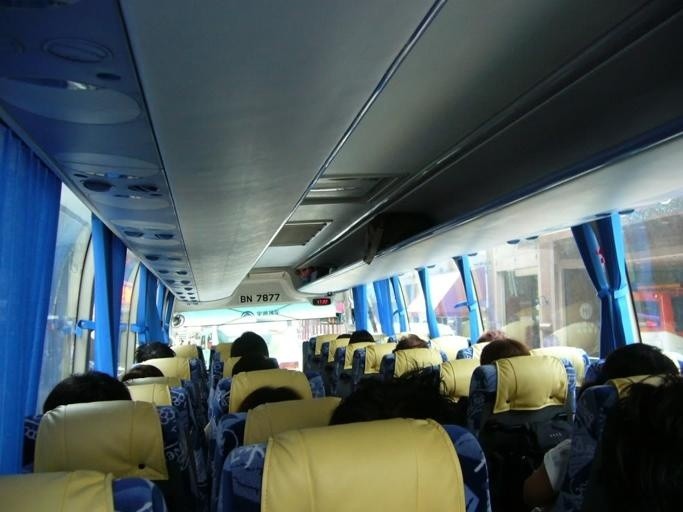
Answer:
[360,212,432,265]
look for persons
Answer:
[477,329,510,342]
[583,374,682,511]
[517,342,674,511]
[480,337,530,366]
[41,329,468,429]
